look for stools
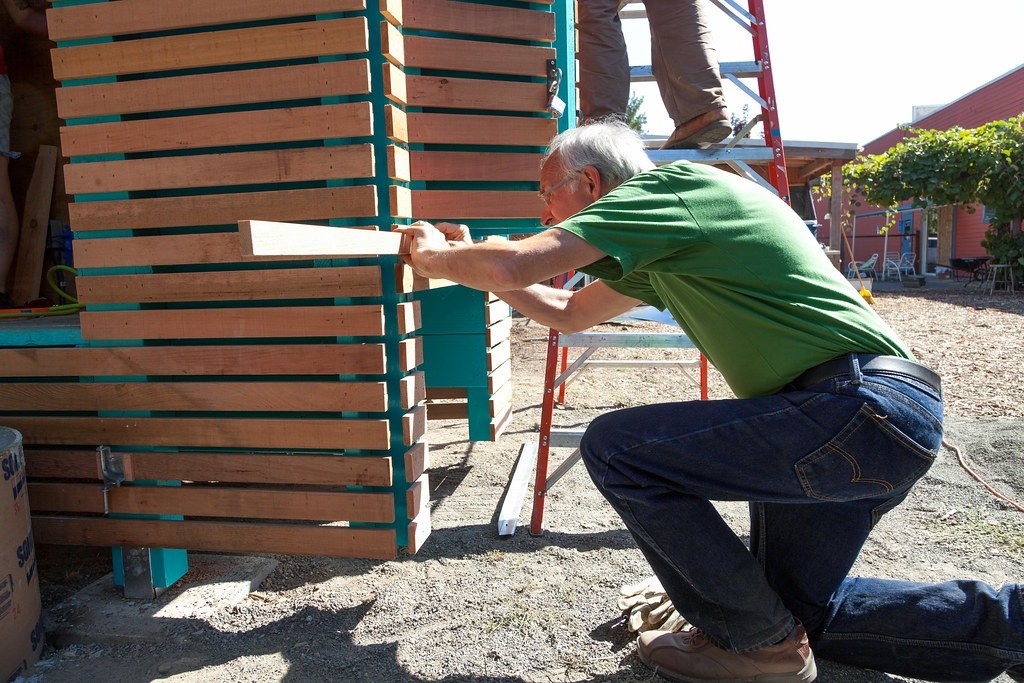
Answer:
[983,264,1014,297]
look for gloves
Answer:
[616,572,697,635]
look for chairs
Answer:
[847,251,917,283]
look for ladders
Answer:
[528,0,789,538]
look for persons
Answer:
[578,0,732,150]
[0,0,48,308]
[390,120,1024,683]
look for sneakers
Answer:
[634,617,819,683]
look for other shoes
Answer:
[657,107,734,150]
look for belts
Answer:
[791,348,943,398]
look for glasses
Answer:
[538,172,576,208]
